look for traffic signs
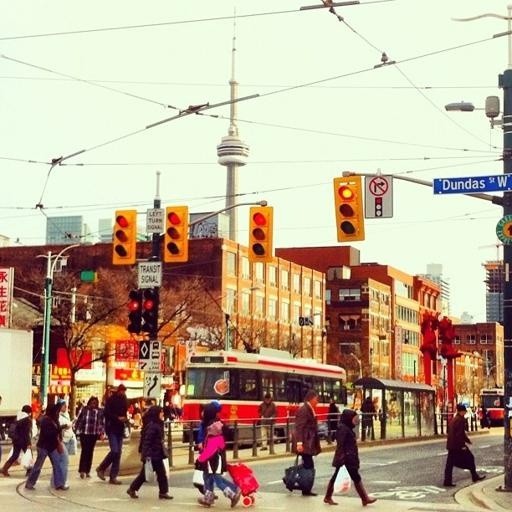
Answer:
[433,172,512,195]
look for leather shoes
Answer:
[56,486,69,490]
[25,486,35,490]
[80,468,121,484]
[473,476,486,483]
[159,493,173,499]
[302,493,317,496]
[126,488,139,498]
[443,483,456,487]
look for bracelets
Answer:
[297,442,303,445]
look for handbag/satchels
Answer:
[454,445,473,468]
[122,420,130,438]
[283,464,316,490]
[193,469,205,485]
[64,437,76,454]
[154,458,170,481]
[72,418,82,435]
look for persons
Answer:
[258,395,276,451]
[283,390,321,495]
[327,399,340,445]
[130,399,180,430]
[194,419,241,507]
[443,403,486,486]
[325,409,377,506]
[25,404,70,492]
[96,383,129,484]
[8,396,73,466]
[0,405,33,477]
[196,402,222,453]
[73,396,105,479]
[465,405,490,431]
[127,406,175,501]
[360,396,375,442]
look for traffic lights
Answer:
[375,197,383,217]
[332,173,366,242]
[248,205,274,265]
[162,203,190,264]
[111,209,137,266]
[142,289,160,341]
[128,288,144,338]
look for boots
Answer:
[0,462,11,476]
[354,479,377,506]
[198,490,214,508]
[324,482,339,505]
[223,487,240,507]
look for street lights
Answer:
[38,240,97,416]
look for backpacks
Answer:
[8,423,21,441]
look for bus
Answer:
[479,386,506,428]
[180,347,350,450]
